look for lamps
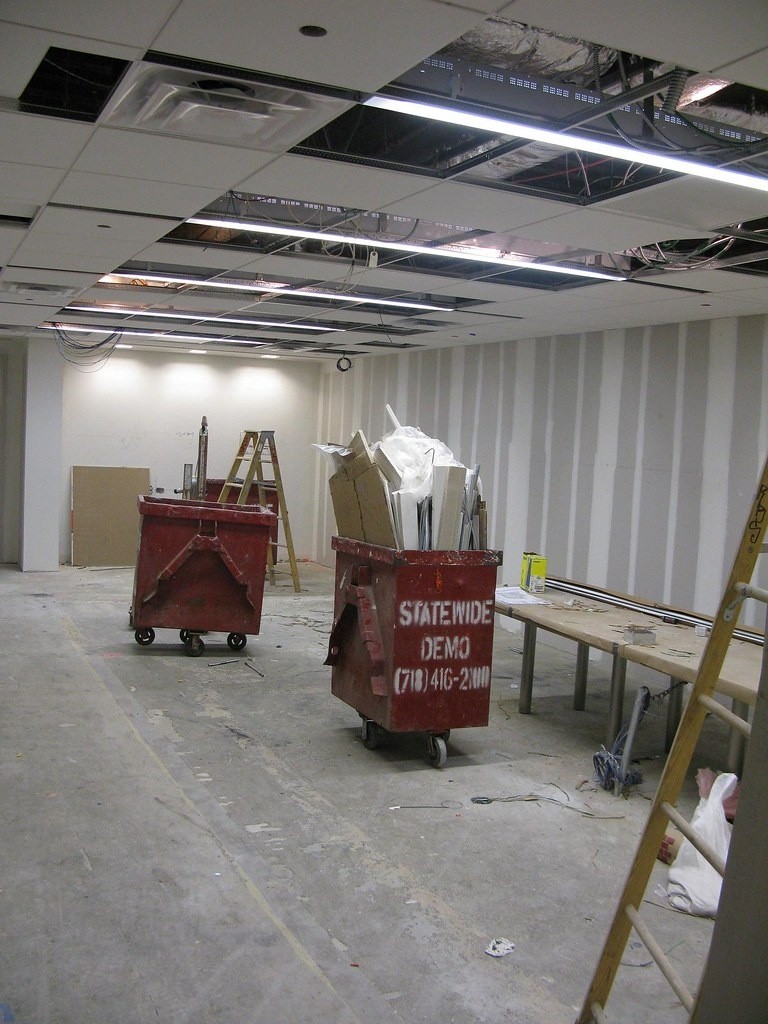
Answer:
[188,215,627,282]
[37,321,275,346]
[63,300,348,333]
[361,89,768,192]
[111,266,455,311]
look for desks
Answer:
[494,584,765,780]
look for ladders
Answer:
[576,444,768,1024]
[217,430,301,593]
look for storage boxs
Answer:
[521,552,548,593]
